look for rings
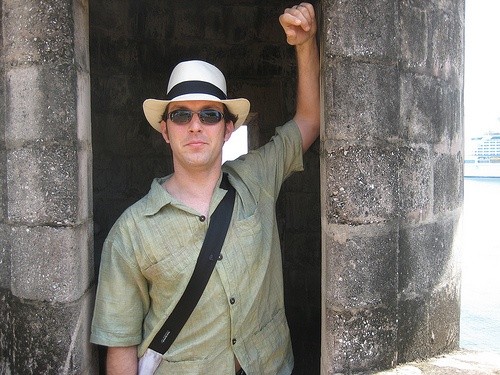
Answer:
[294,5,302,9]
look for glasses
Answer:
[165,108,226,125]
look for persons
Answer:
[89,3,320,375]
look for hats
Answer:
[142,59,251,134]
